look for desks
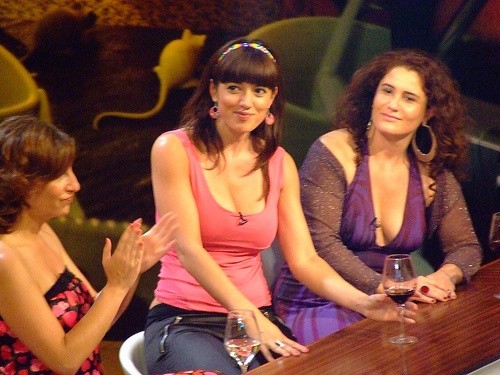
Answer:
[240,259,500,375]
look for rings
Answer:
[276,340,285,348]
[419,285,430,295]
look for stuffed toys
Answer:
[85,29,207,133]
[17,10,96,67]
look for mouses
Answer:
[91,27,208,129]
[19,9,98,63]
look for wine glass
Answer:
[382,254,418,344]
[223,309,262,375]
[488,212,500,298]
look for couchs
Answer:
[0,16,239,226]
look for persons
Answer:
[271,47,484,346]
[0,115,225,375]
[142,38,417,375]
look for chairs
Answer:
[245,16,393,170]
[0,44,50,122]
